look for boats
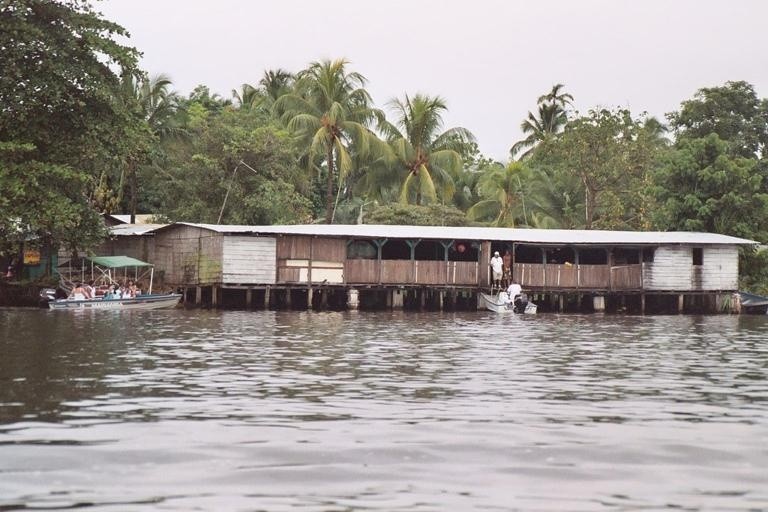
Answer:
[479,292,537,315]
[49,255,184,312]
[739,290,768,313]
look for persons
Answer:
[507,280,522,306]
[6,264,13,282]
[490,251,503,289]
[503,250,513,289]
[498,290,508,303]
[67,280,136,300]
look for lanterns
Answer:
[457,244,465,253]
